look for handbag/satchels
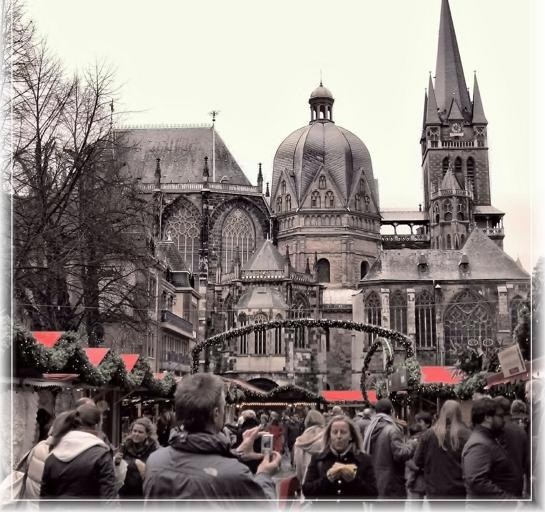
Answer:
[3,468,26,506]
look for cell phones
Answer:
[261,433,273,455]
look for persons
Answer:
[14,371,542,508]
